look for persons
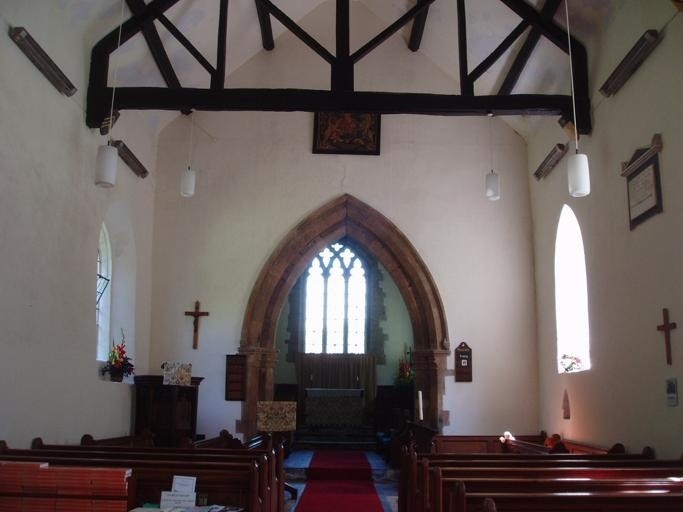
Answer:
[318,109,376,149]
[550,433,568,453]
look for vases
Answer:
[112,373,123,381]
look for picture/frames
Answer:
[624,158,662,231]
[311,112,382,156]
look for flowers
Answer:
[99,327,135,377]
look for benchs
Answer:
[1,433,286,510]
[411,447,683,512]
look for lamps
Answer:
[8,26,77,97]
[483,0,592,199]
[95,0,198,200]
[598,28,660,98]
